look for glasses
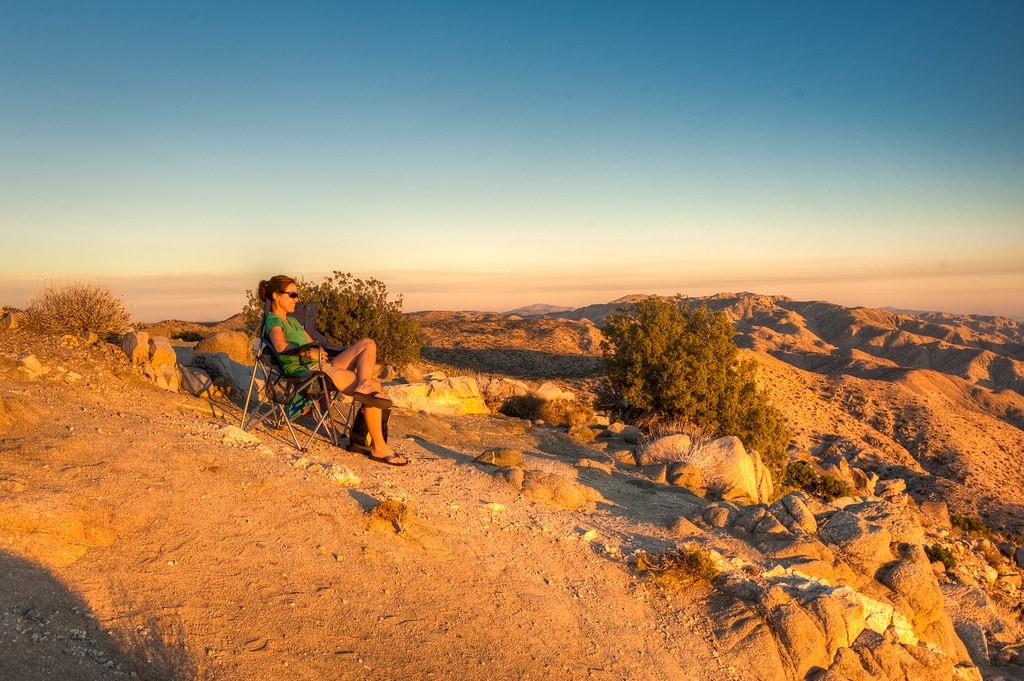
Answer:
[277,291,298,299]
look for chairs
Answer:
[240,299,355,453]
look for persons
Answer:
[258,275,408,466]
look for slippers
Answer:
[370,452,408,466]
[353,392,392,410]
